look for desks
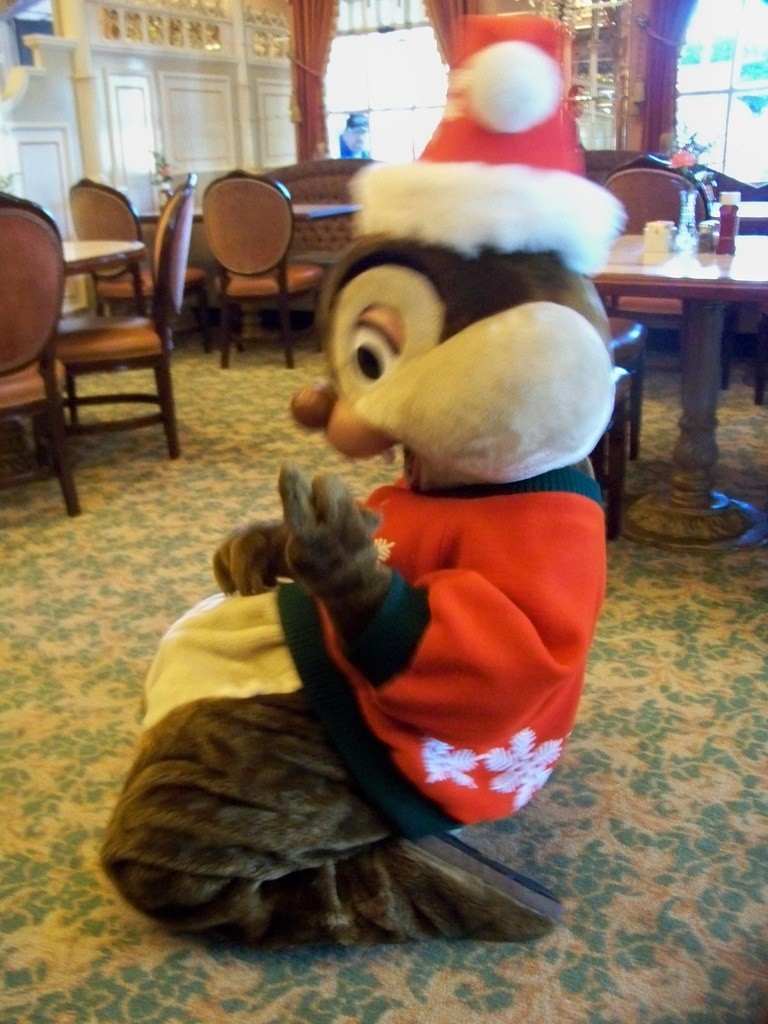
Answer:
[593,234,768,555]
[136,203,357,349]
[712,199,768,229]
[0,238,145,473]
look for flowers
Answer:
[152,151,175,178]
[668,131,720,190]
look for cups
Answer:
[643,220,677,254]
[721,191,741,205]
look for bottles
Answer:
[698,205,739,256]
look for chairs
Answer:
[68,178,213,355]
[59,171,200,460]
[205,168,325,371]
[0,191,81,517]
[600,314,652,539]
[597,153,712,333]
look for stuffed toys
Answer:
[98,17,628,949]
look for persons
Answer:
[336,114,370,159]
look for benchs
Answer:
[263,157,375,263]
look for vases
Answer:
[674,184,699,252]
[158,182,172,211]
